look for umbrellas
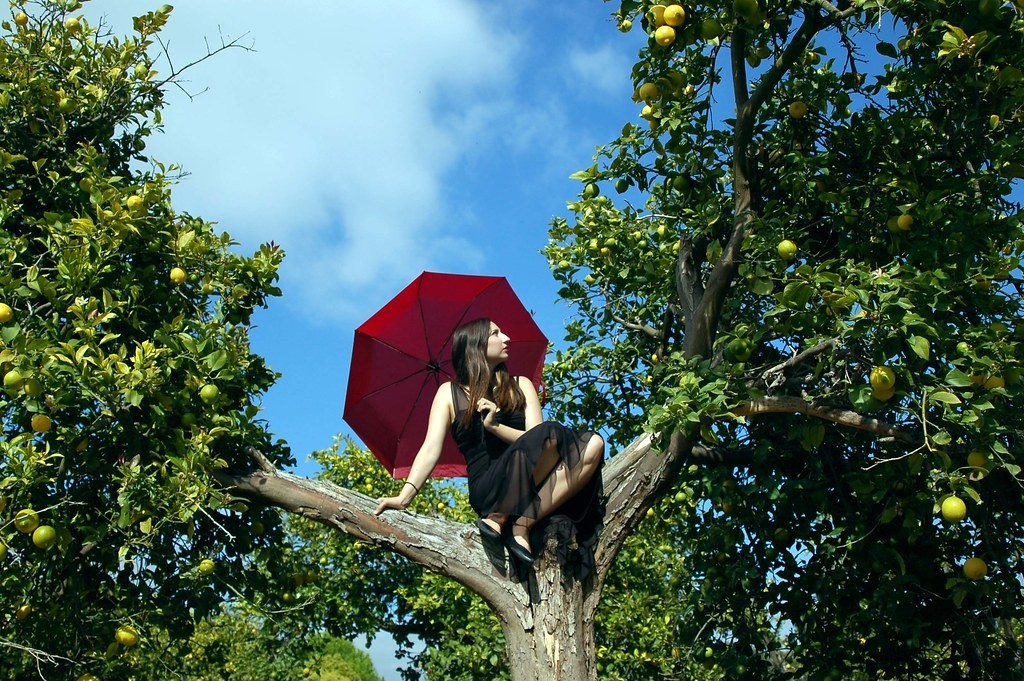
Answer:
[341,271,550,480]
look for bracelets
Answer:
[405,482,420,494]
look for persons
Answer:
[374,318,605,601]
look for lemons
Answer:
[282,468,445,620]
[557,5,1004,583]
[0,10,221,681]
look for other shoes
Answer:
[474,515,502,540]
[504,517,535,564]
